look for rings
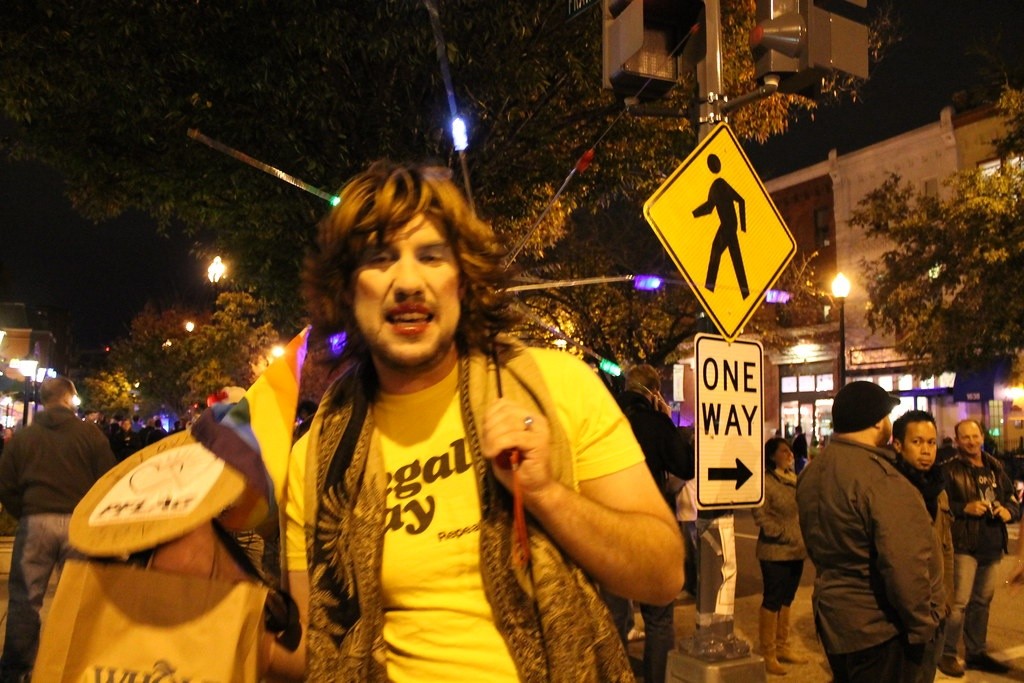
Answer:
[523,416,533,431]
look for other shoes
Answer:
[964,652,1011,675]
[938,654,965,676]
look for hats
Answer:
[833,381,901,434]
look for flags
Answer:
[220,325,308,532]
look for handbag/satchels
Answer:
[33,529,269,683]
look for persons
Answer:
[889,409,954,667]
[937,433,1024,524]
[774,426,808,474]
[752,438,808,674]
[294,400,317,446]
[794,381,949,683]
[84,410,188,465]
[938,419,1020,678]
[596,365,699,683]
[0,376,117,683]
[152,160,684,683]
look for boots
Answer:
[757,605,789,675]
[777,605,809,665]
